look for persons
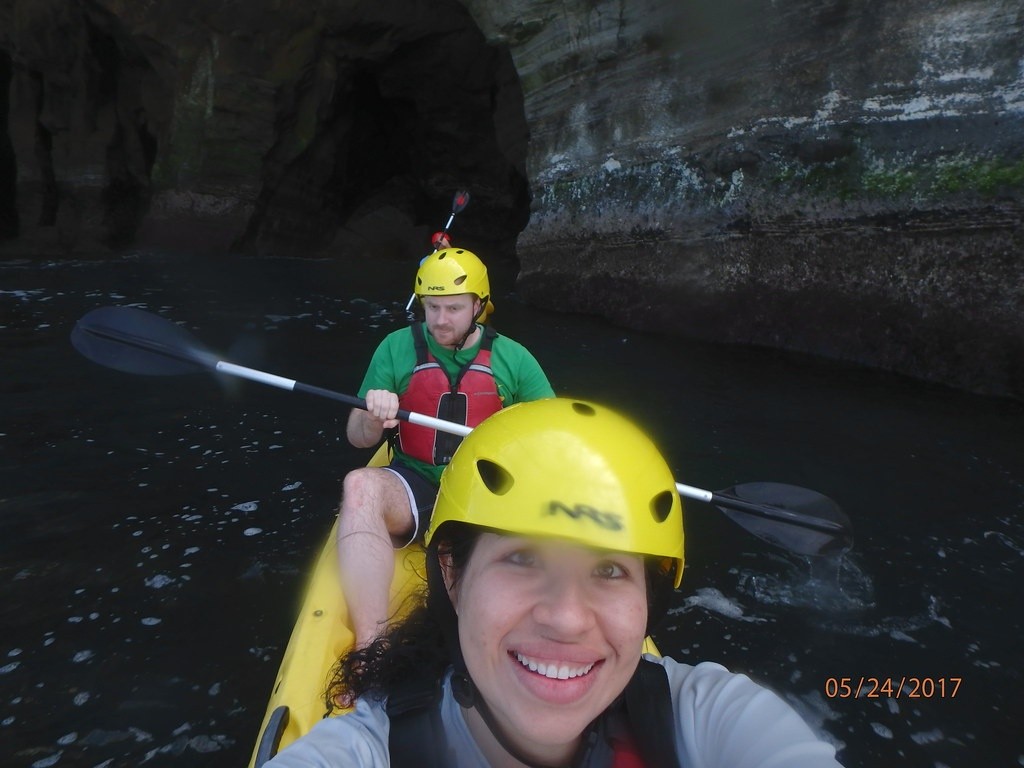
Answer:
[332,232,558,709]
[263,399,853,768]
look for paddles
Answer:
[403,185,471,311]
[69,304,855,559]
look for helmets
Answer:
[415,248,490,301]
[424,399,685,589]
[432,232,451,244]
